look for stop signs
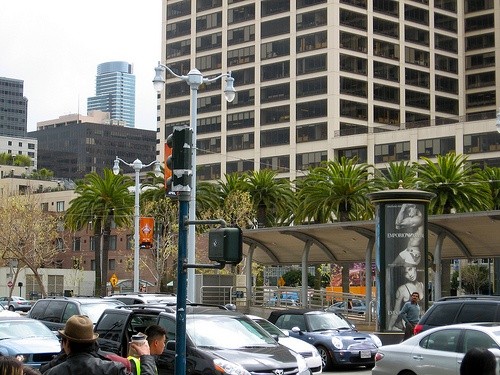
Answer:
[7,282,12,287]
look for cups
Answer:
[132,335,146,357]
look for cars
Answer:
[269,309,382,372]
[0,317,62,369]
[268,292,300,306]
[0,305,20,317]
[371,322,500,375]
[0,297,32,312]
[325,299,365,314]
[247,314,323,375]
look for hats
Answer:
[57,315,99,340]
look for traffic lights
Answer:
[166,124,191,201]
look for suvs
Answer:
[101,292,190,309]
[412,295,500,336]
[27,295,129,340]
[95,302,313,375]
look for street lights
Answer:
[111,156,161,293]
[151,61,237,375]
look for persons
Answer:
[460,348,496,375]
[392,204,424,264]
[399,291,421,341]
[389,267,424,331]
[0,314,167,375]
